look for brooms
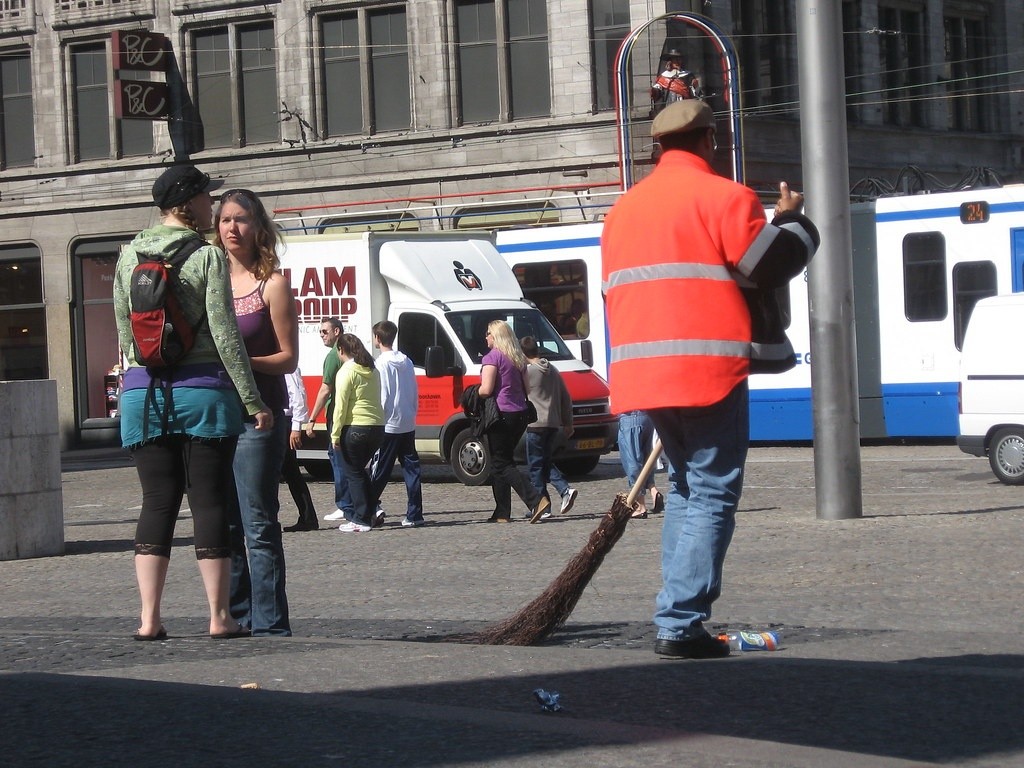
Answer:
[473,190,795,646]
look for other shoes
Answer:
[284,517,319,532]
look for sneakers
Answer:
[401,517,425,526]
[376,506,385,528]
[338,521,372,532]
[526,512,552,518]
[561,487,578,514]
[323,509,349,520]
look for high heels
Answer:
[531,496,551,524]
[488,513,510,523]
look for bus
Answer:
[494,180,1024,441]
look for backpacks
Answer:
[131,255,197,369]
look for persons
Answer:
[306,318,424,532]
[600,99,821,659]
[114,165,274,641]
[520,336,578,519]
[618,410,664,519]
[651,49,698,107]
[460,320,551,524]
[266,367,319,532]
[212,189,299,637]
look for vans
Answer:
[957,292,1023,488]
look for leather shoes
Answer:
[652,492,663,514]
[654,630,730,658]
[629,511,647,519]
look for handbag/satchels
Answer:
[525,401,537,424]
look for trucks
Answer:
[118,230,620,490]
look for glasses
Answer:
[712,134,718,151]
[320,330,328,334]
[220,188,259,204]
[485,332,490,337]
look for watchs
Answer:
[309,419,314,423]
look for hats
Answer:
[659,48,688,61]
[651,99,717,140]
[151,164,225,210]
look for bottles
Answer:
[718,630,780,652]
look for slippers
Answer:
[134,625,167,640]
[211,622,250,638]
